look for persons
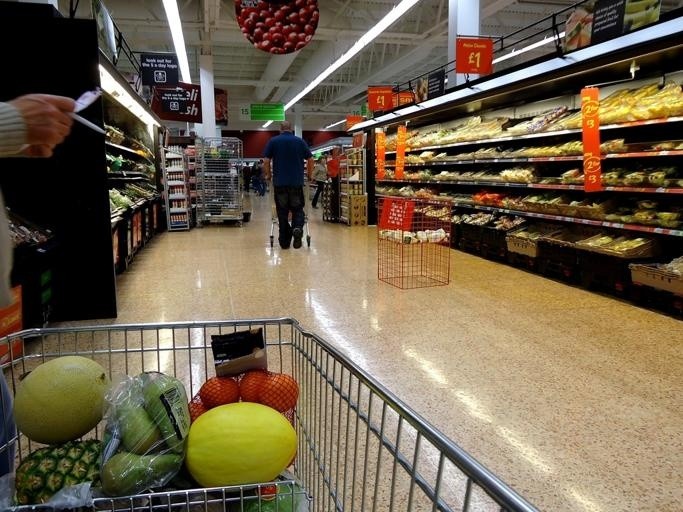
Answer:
[0,93,75,477]
[264,121,313,250]
[242,159,266,196]
[311,156,327,210]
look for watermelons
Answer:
[230,469,305,511]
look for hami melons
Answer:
[187,401,298,490]
[12,356,114,445]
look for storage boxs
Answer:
[378,195,451,288]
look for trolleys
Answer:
[270,182,311,247]
[0,316,542,512]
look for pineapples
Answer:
[14,440,105,509]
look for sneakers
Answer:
[281,225,292,249]
[292,226,303,249]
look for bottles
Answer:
[172,200,190,209]
[168,172,189,182]
[168,145,185,154]
[168,159,188,169]
[169,185,190,194]
[171,214,193,224]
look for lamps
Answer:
[446,32,565,83]
[262,0,417,129]
[324,119,347,129]
[163,0,192,85]
[99,64,160,128]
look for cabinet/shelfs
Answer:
[304,137,353,188]
[339,148,367,225]
[187,149,198,212]
[4,3,161,318]
[163,148,191,231]
[362,0,682,320]
